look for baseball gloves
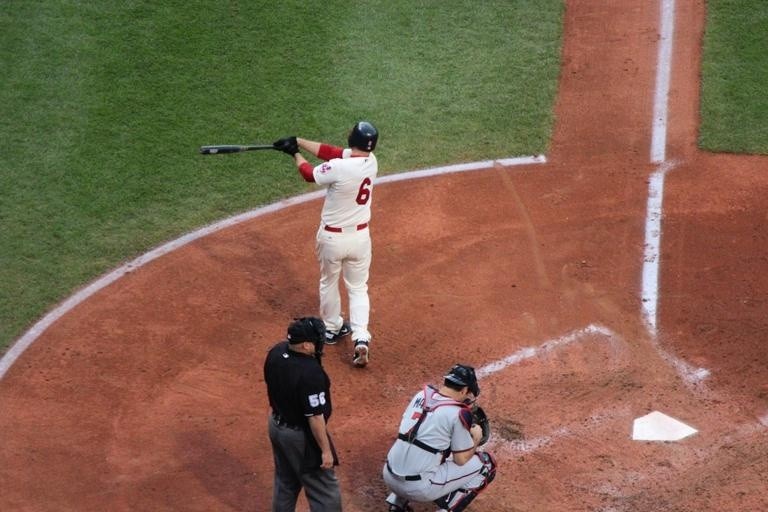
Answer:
[471,406,489,446]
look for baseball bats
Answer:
[201,145,274,154]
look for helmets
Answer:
[287,317,325,344]
[443,365,476,385]
[349,122,377,151]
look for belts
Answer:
[386,461,421,481]
[272,412,302,430]
[325,223,367,232]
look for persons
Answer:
[383,363,497,512]
[263,317,342,512]
[273,121,379,368]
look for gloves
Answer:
[274,136,300,156]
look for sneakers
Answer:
[325,324,368,368]
